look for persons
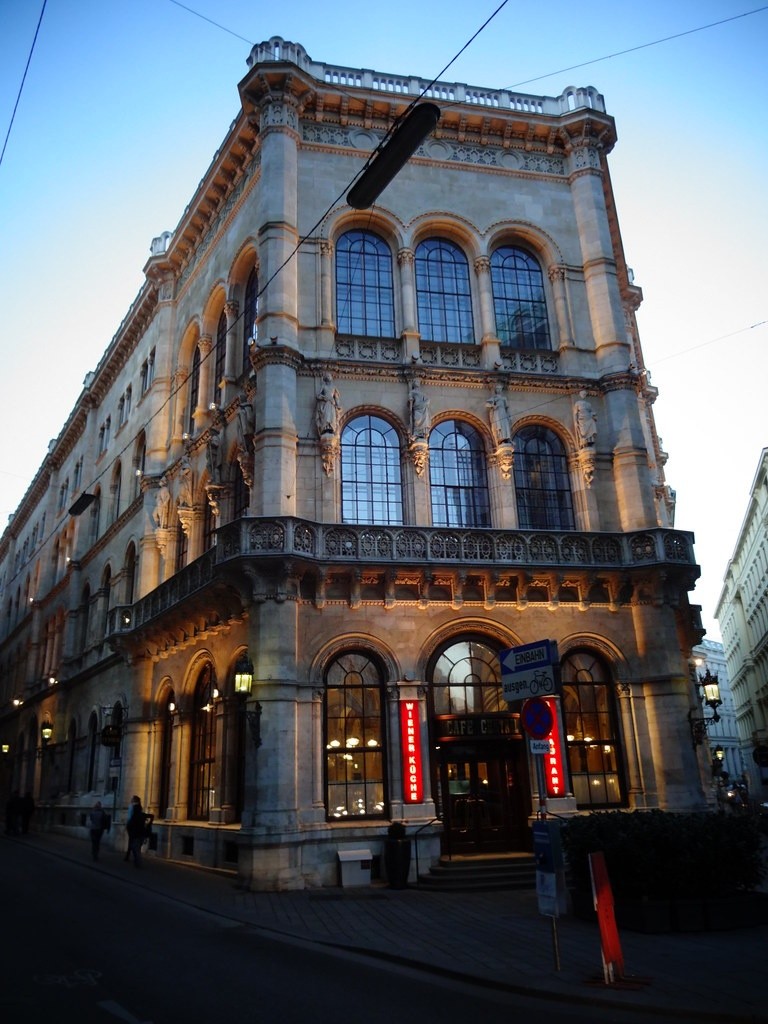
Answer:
[89,801,107,862]
[483,385,512,445]
[572,391,598,448]
[316,374,342,439]
[4,790,36,837]
[152,394,256,526]
[124,795,154,868]
[408,379,430,438]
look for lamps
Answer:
[494,359,502,369]
[233,651,263,749]
[686,665,723,751]
[248,338,259,348]
[412,352,419,363]
[40,721,59,758]
[218,380,226,389]
[628,361,636,371]
[270,335,278,345]
[710,743,724,760]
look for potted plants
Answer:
[383,822,413,892]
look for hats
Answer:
[95,801,100,806]
[130,796,140,803]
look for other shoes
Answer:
[123,857,129,862]
[134,860,141,868]
[91,852,100,862]
